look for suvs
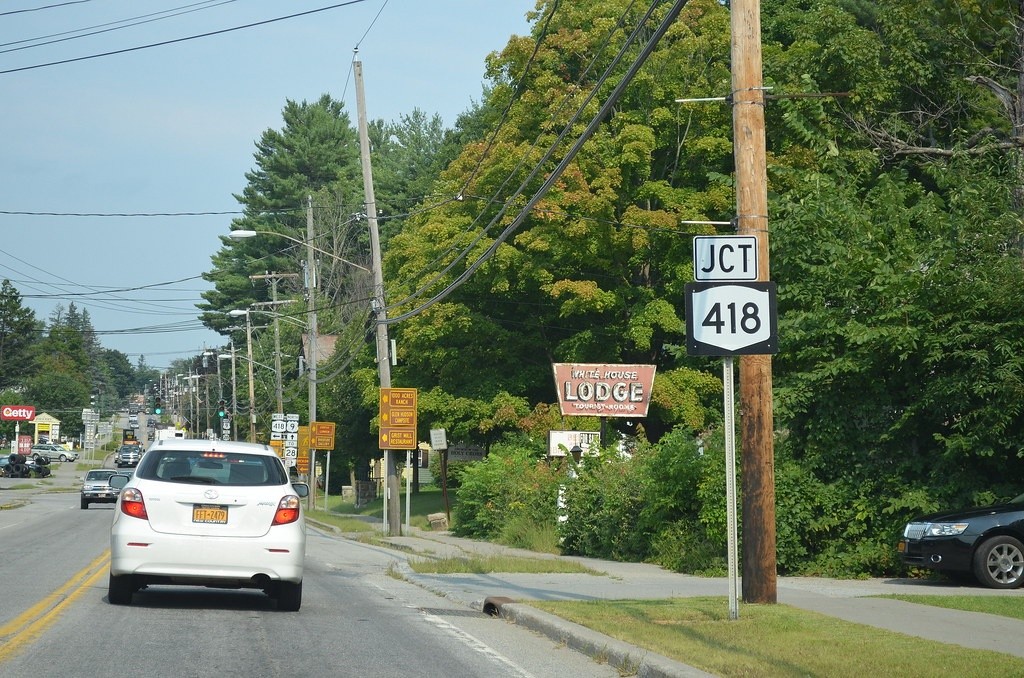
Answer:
[78,469,120,510]
[118,445,142,468]
[30,444,79,463]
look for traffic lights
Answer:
[218,399,225,417]
[155,396,162,415]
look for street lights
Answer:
[175,372,238,442]
[229,230,402,538]
[217,353,282,417]
[227,310,317,513]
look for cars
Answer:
[114,402,184,462]
[898,493,1024,590]
[107,438,309,612]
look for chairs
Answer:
[163,459,191,480]
[229,464,264,483]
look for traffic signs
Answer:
[380,407,418,427]
[379,426,417,450]
[379,388,417,409]
[308,436,334,450]
[309,421,336,436]
